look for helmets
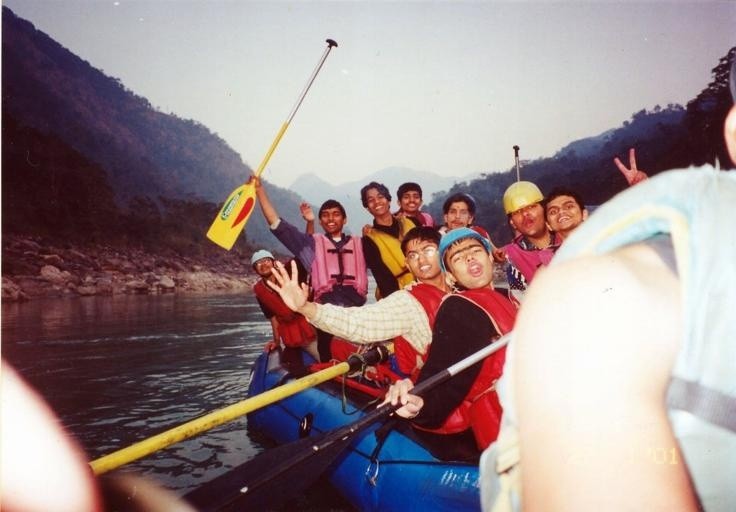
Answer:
[439,226,491,272]
[503,181,544,215]
[251,250,275,266]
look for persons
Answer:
[503,180,561,291]
[266,225,454,376]
[250,203,321,362]
[249,175,371,362]
[493,63,735,512]
[361,182,425,300]
[375,226,521,452]
[493,148,648,264]
[437,192,491,240]
[393,182,434,228]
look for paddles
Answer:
[180,330,511,512]
[207,38,337,251]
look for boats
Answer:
[239,341,483,512]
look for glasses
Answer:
[404,246,437,263]
